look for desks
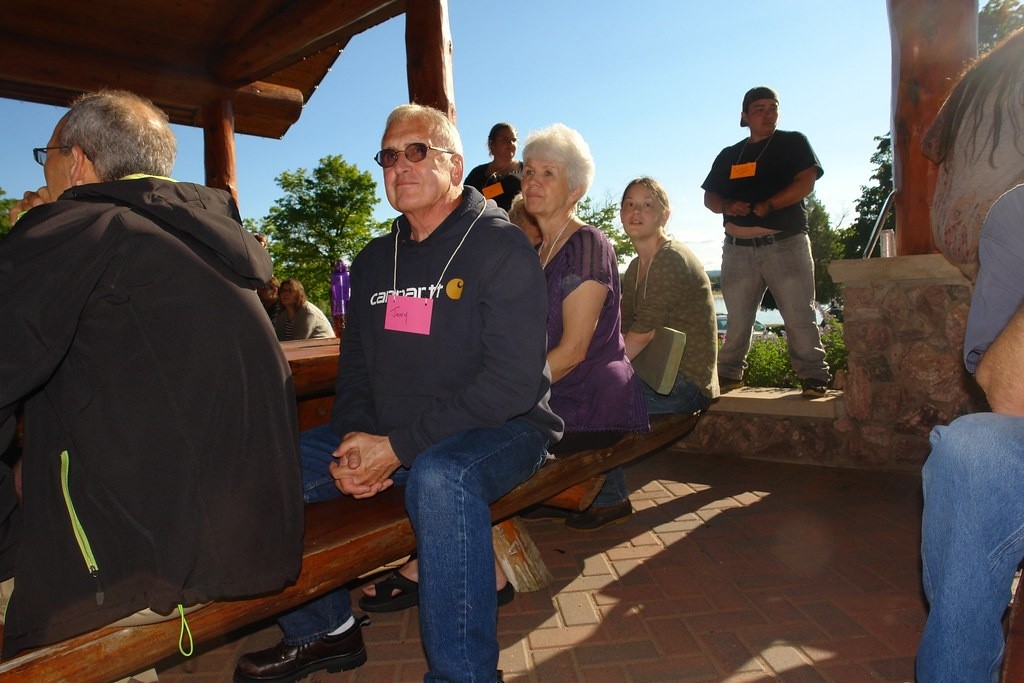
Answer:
[281,334,344,404]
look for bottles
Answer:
[880,228,896,258]
[331,260,351,337]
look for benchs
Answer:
[0,405,708,683]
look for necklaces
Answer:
[539,215,573,269]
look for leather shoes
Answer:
[519,507,575,523]
[566,499,633,531]
[233,614,371,683]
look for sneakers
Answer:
[717,375,745,390]
[799,379,829,396]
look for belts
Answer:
[726,229,802,246]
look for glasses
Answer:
[374,144,454,167]
[33,146,76,165]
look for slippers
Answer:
[494,581,515,604]
[358,571,421,611]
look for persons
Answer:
[510,193,543,246]
[520,178,719,529]
[253,233,336,341]
[915,185,1024,683]
[0,91,307,658]
[701,86,832,394]
[463,124,524,213]
[234,106,564,683]
[358,125,649,609]
[921,28,1024,280]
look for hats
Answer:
[739,87,779,127]
